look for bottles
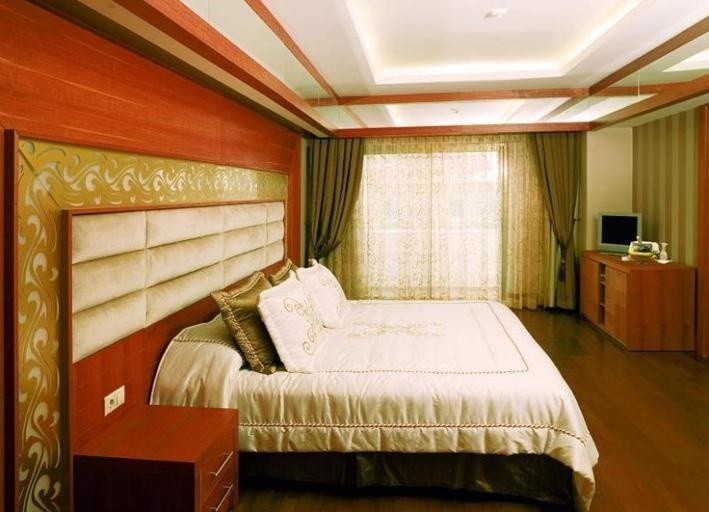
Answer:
[659,244,668,260]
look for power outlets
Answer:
[104,385,126,416]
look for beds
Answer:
[60,198,598,512]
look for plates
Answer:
[656,260,672,264]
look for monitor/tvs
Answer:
[598,212,642,256]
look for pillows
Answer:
[211,271,278,374]
[258,270,331,372]
[269,258,299,286]
[295,258,351,329]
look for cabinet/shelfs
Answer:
[580,249,696,356]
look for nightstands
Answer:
[74,403,239,511]
